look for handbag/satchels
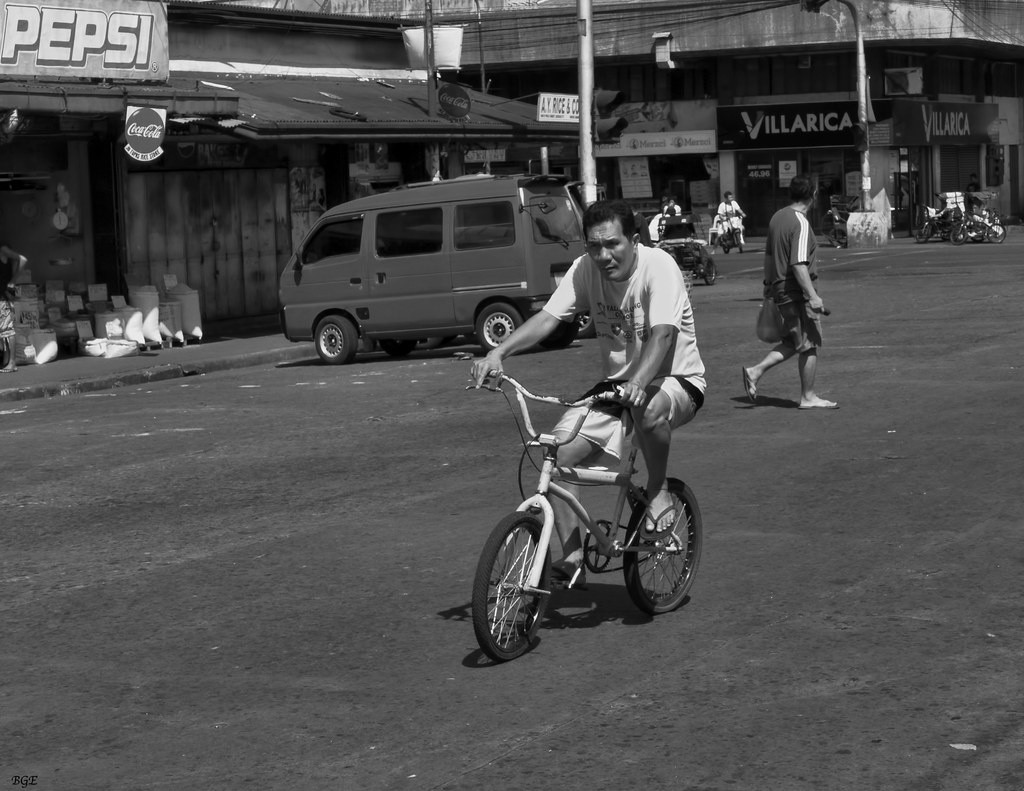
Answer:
[757,297,782,343]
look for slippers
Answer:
[551,566,582,589]
[640,495,677,540]
[743,366,756,402]
[798,400,839,409]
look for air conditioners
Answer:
[884,67,923,96]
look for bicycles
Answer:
[824,205,849,247]
[916,191,1007,244]
[455,351,704,664]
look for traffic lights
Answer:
[591,87,629,143]
[852,123,868,153]
[800,0,829,14]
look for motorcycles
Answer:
[656,210,717,285]
[719,214,745,253]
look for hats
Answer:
[666,207,676,214]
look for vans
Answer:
[278,172,588,364]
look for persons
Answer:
[470,199,707,593]
[717,192,747,243]
[966,173,981,210]
[741,175,838,410]
[0,243,28,373]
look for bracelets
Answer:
[742,212,744,215]
